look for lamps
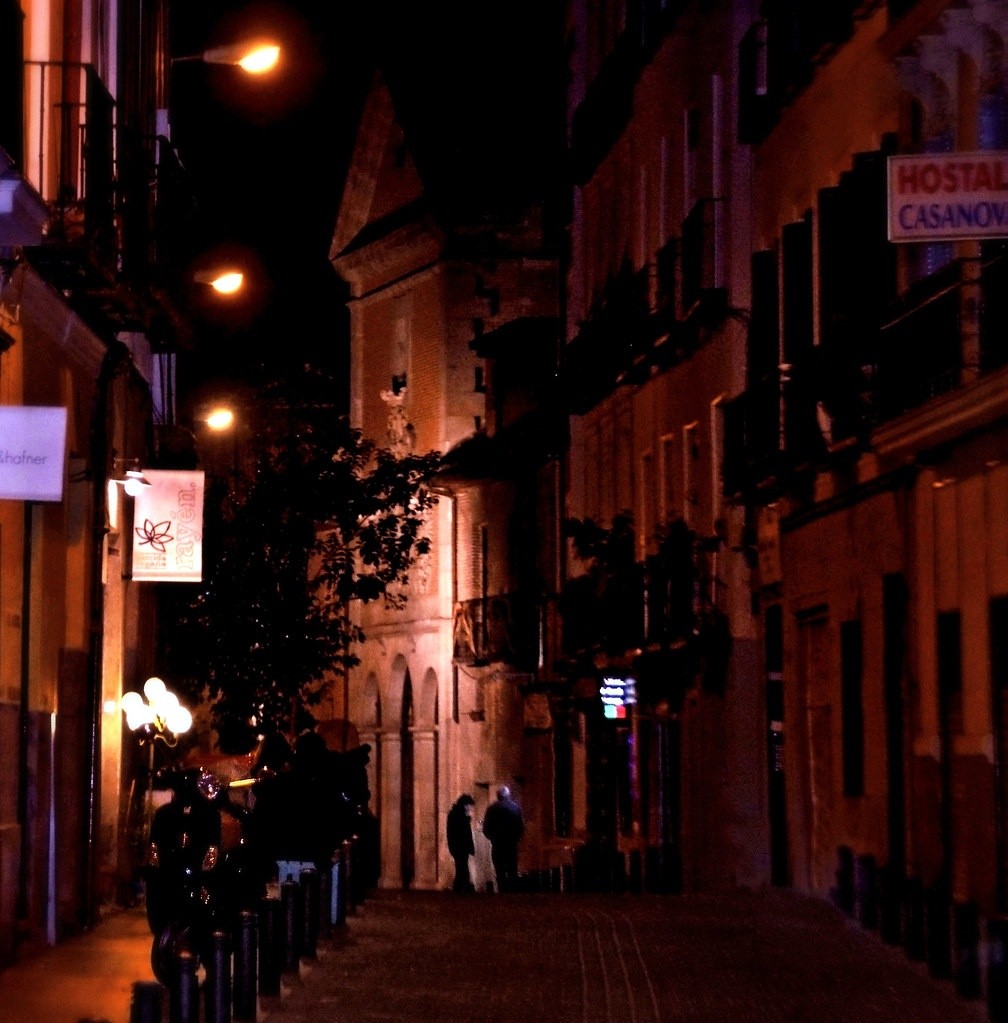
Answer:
[166,30,282,81]
[158,260,247,298]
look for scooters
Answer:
[146,766,221,935]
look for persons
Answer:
[484,788,524,893]
[447,795,476,893]
[147,771,221,967]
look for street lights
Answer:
[145,36,286,275]
[121,676,196,905]
